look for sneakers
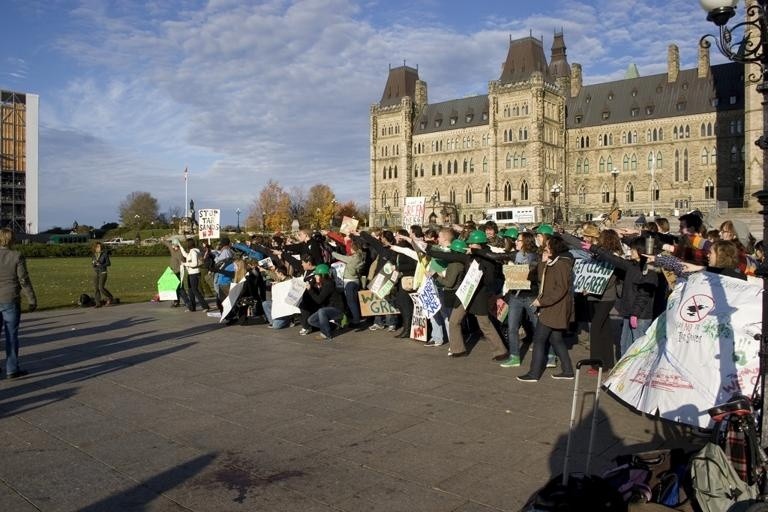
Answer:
[424,340,467,358]
[106,296,114,303]
[171,303,210,312]
[517,373,539,382]
[493,353,521,368]
[528,342,556,368]
[586,367,603,376]
[370,323,385,331]
[6,370,30,379]
[551,372,575,381]
[388,325,396,332]
[266,320,344,342]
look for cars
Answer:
[140,238,161,247]
[589,211,609,222]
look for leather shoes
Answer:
[395,328,411,338]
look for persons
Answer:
[90,241,113,309]
[0,226,37,381]
[157,210,765,383]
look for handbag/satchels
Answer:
[612,449,695,512]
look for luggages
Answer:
[524,470,617,512]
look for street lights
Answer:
[235,206,243,233]
[383,203,391,227]
[172,214,177,234]
[707,178,712,200]
[330,198,338,225]
[315,207,321,230]
[151,221,155,237]
[431,192,437,224]
[611,166,620,204]
[261,210,268,233]
[737,177,741,207]
[550,179,562,227]
[697,0,768,453]
[134,214,141,246]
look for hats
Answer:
[481,219,500,233]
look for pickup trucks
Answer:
[103,237,135,246]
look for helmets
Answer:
[447,239,467,253]
[496,228,519,239]
[314,264,330,274]
[465,230,489,244]
[535,223,554,234]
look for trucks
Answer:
[478,205,544,224]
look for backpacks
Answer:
[689,414,760,512]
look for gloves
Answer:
[581,240,591,249]
[29,304,37,313]
[630,315,637,329]
